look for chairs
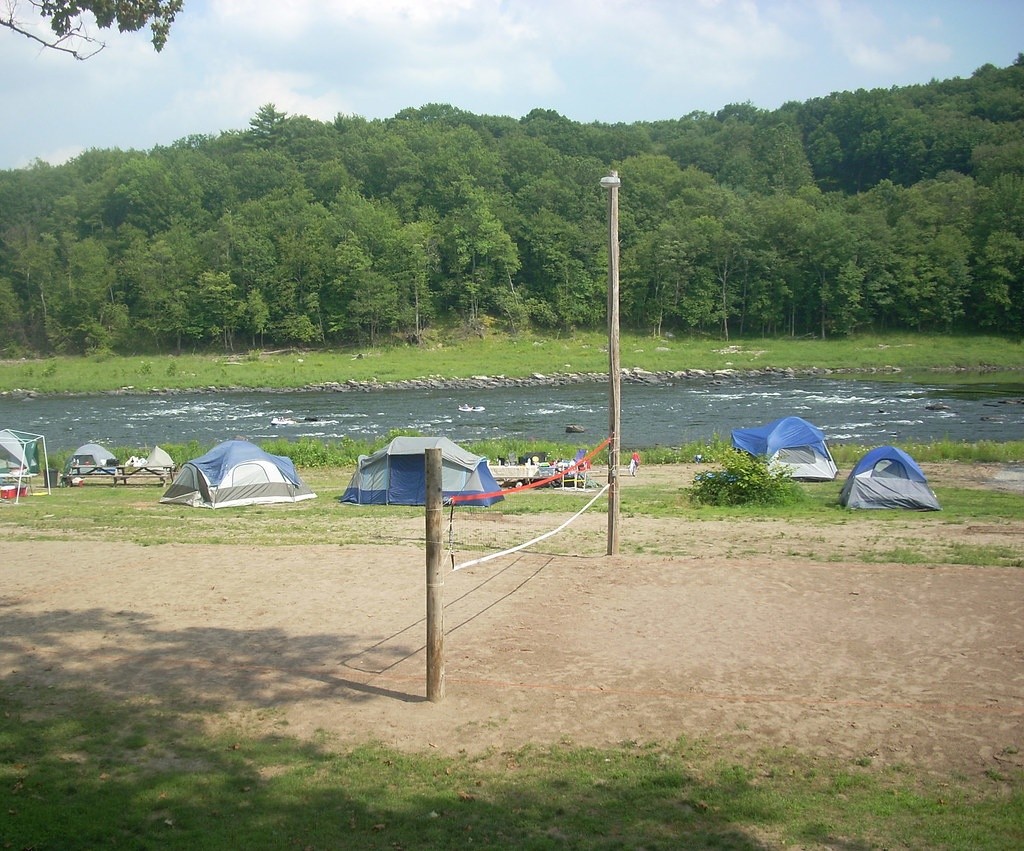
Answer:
[560,462,588,489]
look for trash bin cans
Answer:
[43,468,59,487]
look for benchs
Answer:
[60,472,179,479]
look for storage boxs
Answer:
[0,486,27,499]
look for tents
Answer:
[65,444,117,471]
[839,447,941,511]
[160,441,318,508]
[341,436,505,507]
[731,416,838,482]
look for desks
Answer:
[69,464,174,487]
[488,465,539,485]
